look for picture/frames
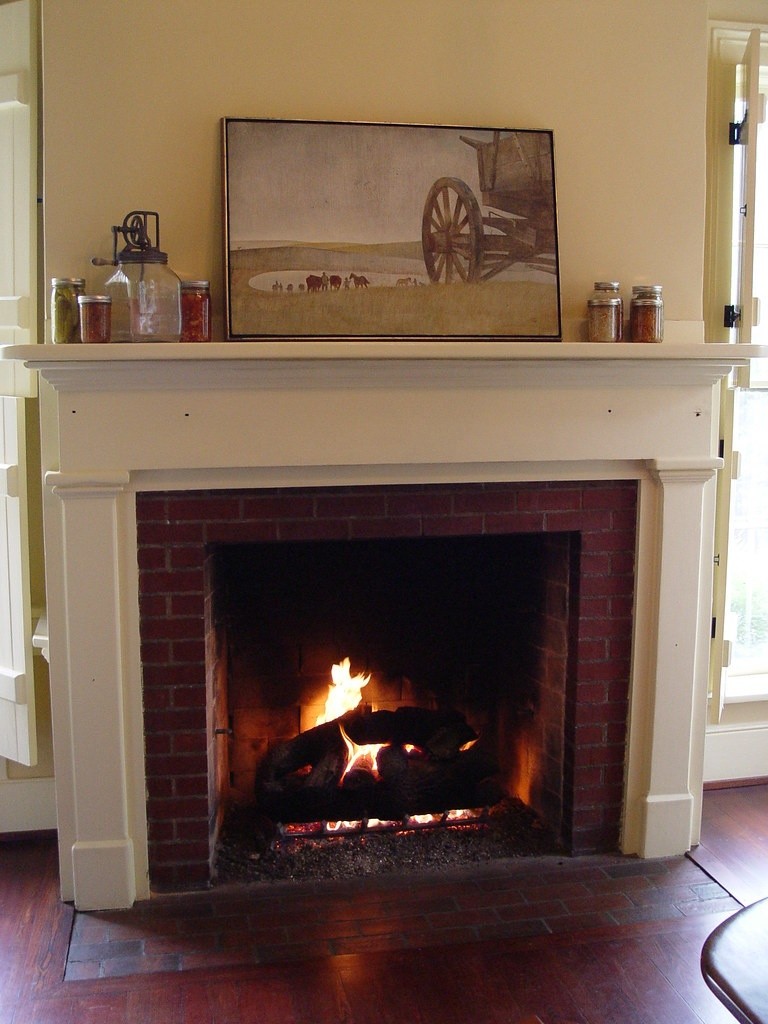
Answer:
[224,116,563,342]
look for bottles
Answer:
[104,251,180,342]
